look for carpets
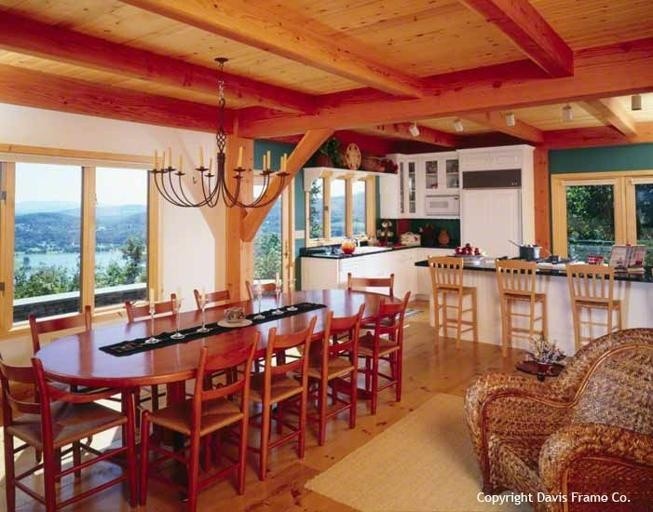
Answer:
[303,392,537,512]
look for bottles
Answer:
[438,229,449,245]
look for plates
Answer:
[216,318,252,328]
[343,143,361,169]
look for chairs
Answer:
[0,272,411,512]
[464,327,653,512]
[495,259,548,358]
[566,263,623,351]
[427,256,479,354]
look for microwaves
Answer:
[425,196,459,215]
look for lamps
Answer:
[148,57,291,208]
[408,93,642,138]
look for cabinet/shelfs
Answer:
[397,152,462,219]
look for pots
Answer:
[508,239,542,261]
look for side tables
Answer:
[515,360,565,382]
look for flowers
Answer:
[525,339,567,365]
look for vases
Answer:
[537,362,548,370]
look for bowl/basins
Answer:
[456,254,486,264]
[224,306,245,323]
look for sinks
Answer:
[352,246,392,255]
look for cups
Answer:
[341,234,360,254]
[587,256,603,264]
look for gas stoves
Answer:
[499,255,576,265]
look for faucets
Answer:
[354,237,361,248]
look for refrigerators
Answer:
[457,188,522,259]
